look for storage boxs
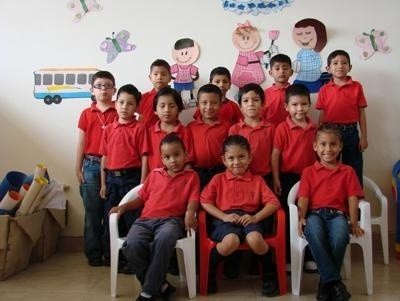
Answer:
[0,200,70,281]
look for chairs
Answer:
[109,175,389,298]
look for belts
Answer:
[338,124,357,130]
[103,167,136,177]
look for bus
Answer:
[33,66,117,104]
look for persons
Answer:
[290,18,327,93]
[230,20,266,88]
[170,38,200,107]
[76,50,369,301]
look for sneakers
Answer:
[135,291,158,301]
[304,260,318,273]
[316,280,330,301]
[207,253,260,293]
[329,278,351,301]
[104,255,136,275]
[261,264,278,297]
[88,256,104,266]
[160,280,177,301]
